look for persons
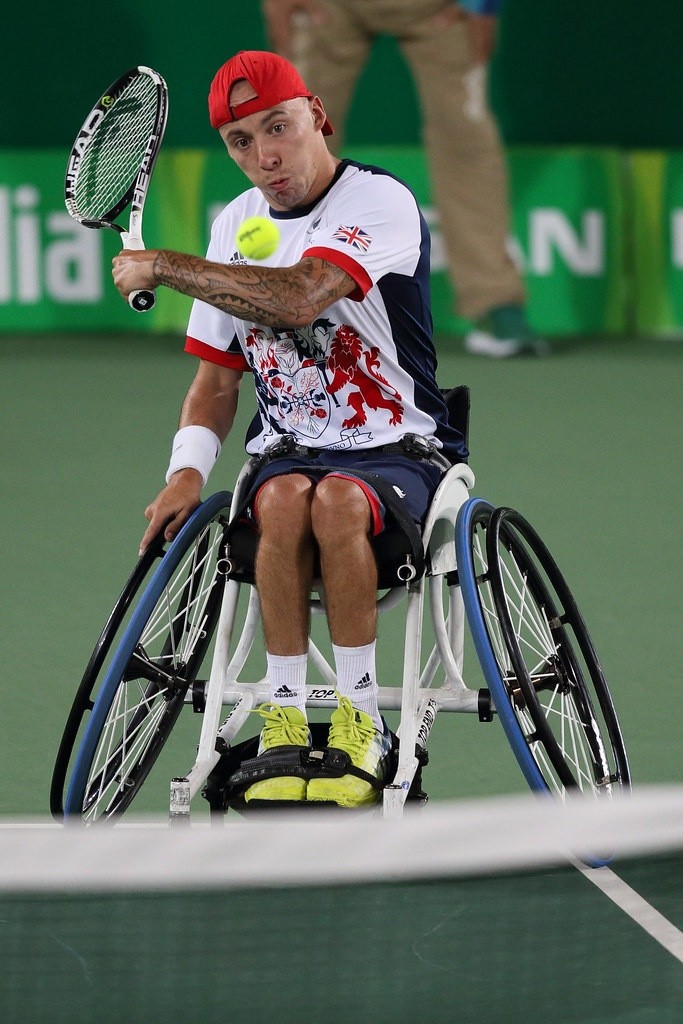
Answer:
[265,0,541,357]
[112,50,469,809]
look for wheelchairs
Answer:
[51,385,631,837]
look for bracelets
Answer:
[165,425,222,490]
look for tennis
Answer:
[234,215,280,260]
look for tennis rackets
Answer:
[63,63,171,315]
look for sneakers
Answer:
[307,682,393,809]
[244,703,312,804]
[462,306,533,359]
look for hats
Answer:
[208,50,334,137]
